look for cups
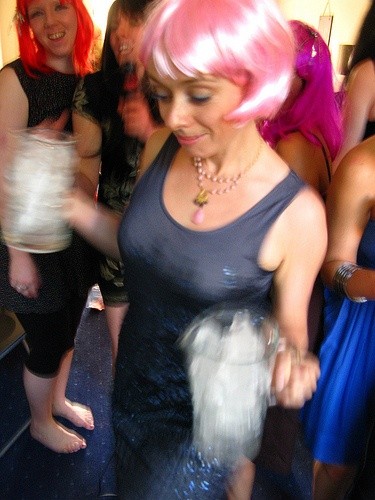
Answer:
[182,302,299,469]
[0,126,82,253]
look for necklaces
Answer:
[191,136,264,224]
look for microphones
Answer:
[123,71,140,154]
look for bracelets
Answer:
[333,264,369,304]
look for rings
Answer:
[15,284,28,294]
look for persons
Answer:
[72,0,164,382]
[308,135,375,500]
[60,0,326,500]
[326,0,375,172]
[258,20,344,459]
[1,0,95,454]
